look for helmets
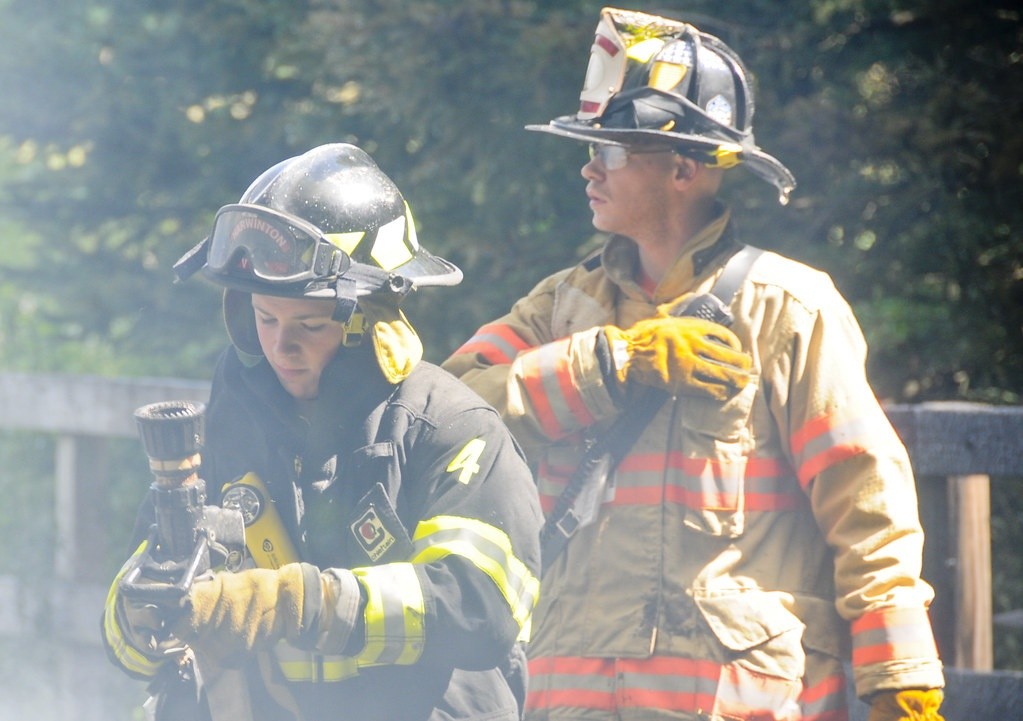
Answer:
[201,143,464,299]
[522,6,795,191]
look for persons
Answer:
[440,7,946,721]
[99,143,547,721]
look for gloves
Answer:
[597,292,750,402]
[170,560,320,669]
[866,687,945,721]
[115,564,182,654]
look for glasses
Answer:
[588,142,676,170]
[207,202,350,282]
